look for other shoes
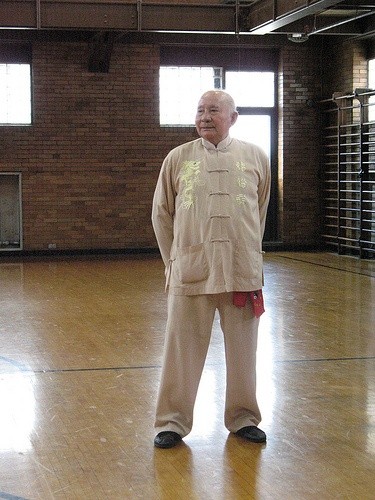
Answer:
[233,426,266,442]
[154,432,181,449]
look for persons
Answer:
[151,90,272,448]
